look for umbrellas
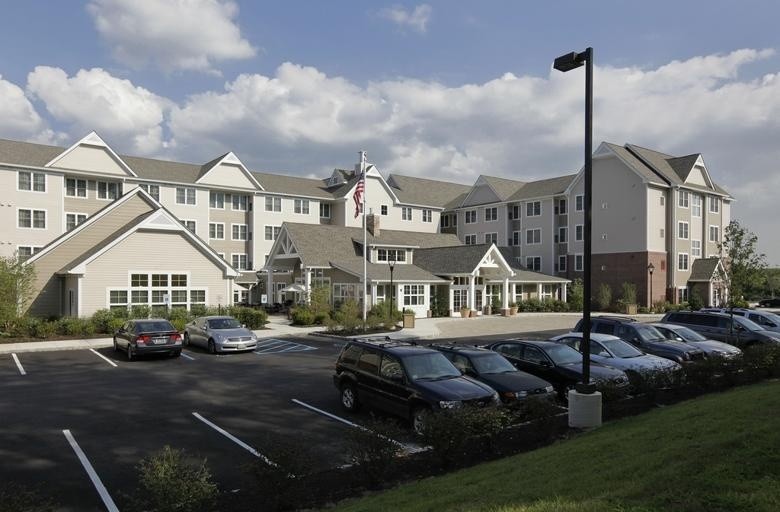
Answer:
[278,281,305,303]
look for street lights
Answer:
[552,46,607,432]
[646,262,656,315]
[386,252,396,331]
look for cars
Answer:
[184,315,258,356]
[476,339,631,408]
[550,331,685,397]
[113,318,185,362]
[642,321,744,379]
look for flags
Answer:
[352,162,365,219]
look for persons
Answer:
[238,300,293,314]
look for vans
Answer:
[697,306,780,336]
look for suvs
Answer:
[659,309,780,376]
[572,315,710,387]
[331,335,505,443]
[421,342,560,407]
